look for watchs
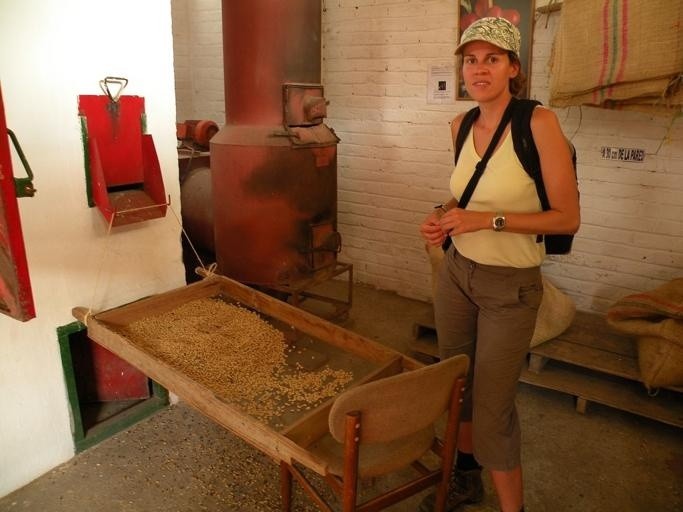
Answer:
[492,208,506,233]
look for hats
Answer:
[454,16,523,57]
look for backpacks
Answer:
[454,100,578,256]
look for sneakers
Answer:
[420,470,485,512]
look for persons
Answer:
[420,14,582,512]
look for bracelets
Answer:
[433,203,447,214]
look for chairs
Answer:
[276,353,470,512]
